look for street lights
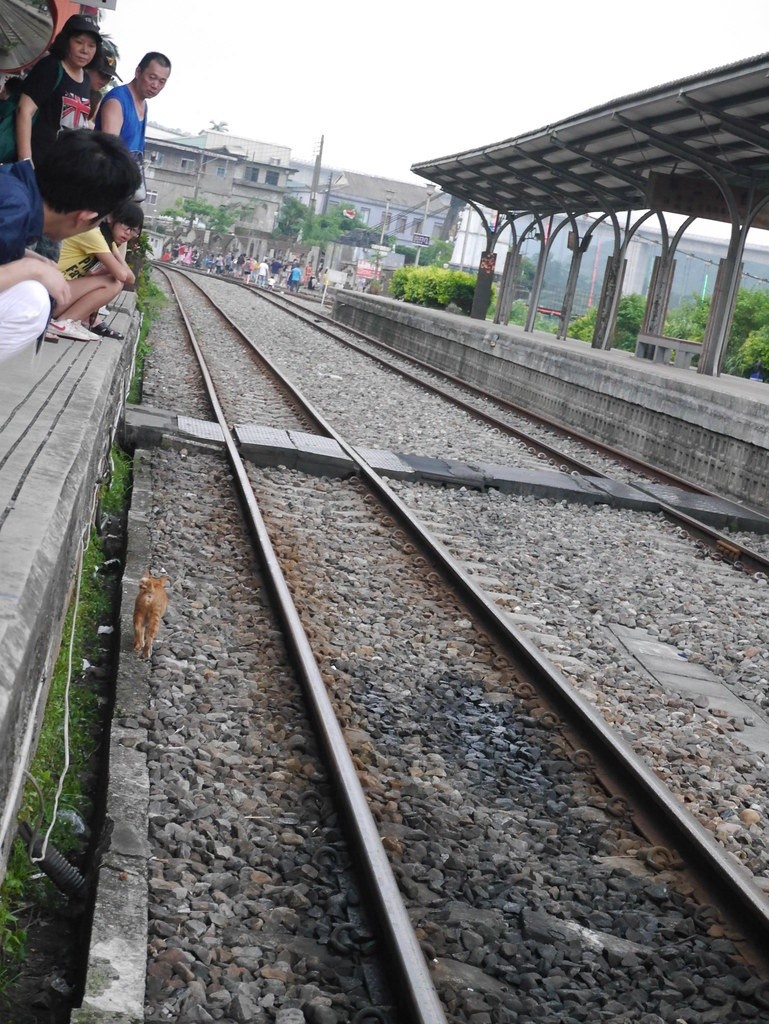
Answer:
[379,189,395,246]
[414,183,436,266]
[273,212,278,229]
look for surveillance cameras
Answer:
[511,212,515,218]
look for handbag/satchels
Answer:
[1,98,40,162]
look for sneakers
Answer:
[46,318,102,343]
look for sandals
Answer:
[92,321,125,341]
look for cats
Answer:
[133,568,169,658]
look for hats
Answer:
[66,13,103,44]
[93,48,123,83]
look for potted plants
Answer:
[387,265,495,316]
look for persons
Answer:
[0,14,170,343]
[171,241,323,292]
[0,129,143,364]
[162,248,172,262]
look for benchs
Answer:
[634,332,703,369]
